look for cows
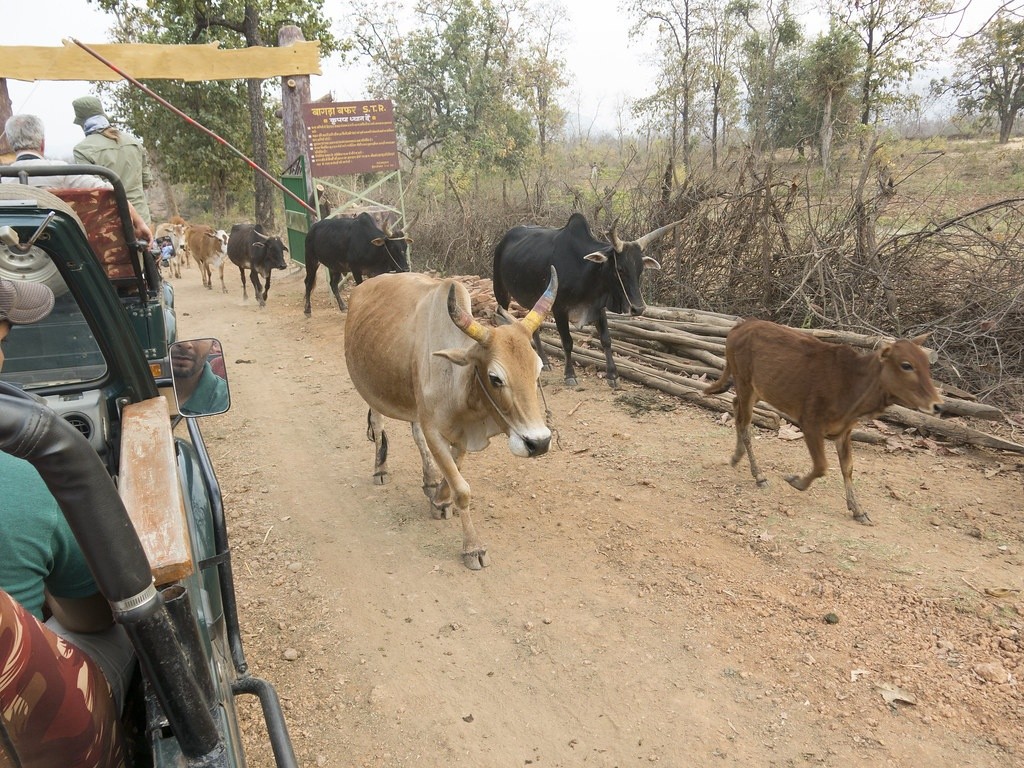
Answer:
[703,318,945,526]
[154,211,691,571]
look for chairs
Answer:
[0,591,143,768]
[50,188,138,279]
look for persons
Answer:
[0,113,154,251]
[72,96,152,272]
[170,340,230,414]
[159,240,175,259]
[0,276,142,723]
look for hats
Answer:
[72,96,109,124]
[0,277,55,325]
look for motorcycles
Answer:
[0,160,177,393]
